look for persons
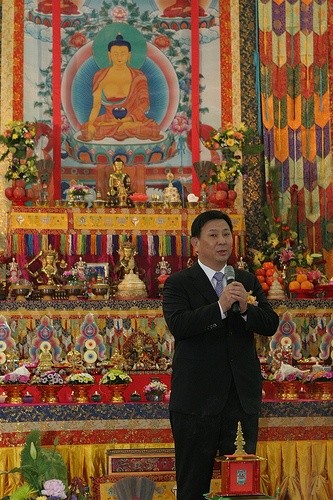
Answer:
[113,242,146,282]
[41,251,57,284]
[39,345,52,366]
[109,158,131,204]
[112,350,125,369]
[163,210,279,500]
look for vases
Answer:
[37,385,62,403]
[107,384,128,403]
[5,180,34,206]
[273,380,301,399]
[66,276,79,285]
[150,390,164,402]
[73,196,84,200]
[313,382,333,400]
[1,384,29,404]
[69,385,94,404]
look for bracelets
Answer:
[241,310,247,316]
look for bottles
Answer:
[130,390,140,401]
[23,391,33,404]
[91,390,101,402]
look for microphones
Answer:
[224,266,240,313]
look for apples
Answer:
[209,182,237,202]
[255,262,284,291]
[4,180,34,199]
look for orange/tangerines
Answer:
[289,274,314,290]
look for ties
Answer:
[214,273,224,297]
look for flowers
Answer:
[36,372,63,385]
[99,369,132,386]
[252,203,321,283]
[146,381,167,392]
[269,369,307,382]
[70,184,89,195]
[303,370,333,383]
[0,120,38,184]
[64,372,95,384]
[206,122,264,184]
[63,270,84,281]
[0,428,85,500]
[0,374,30,384]
